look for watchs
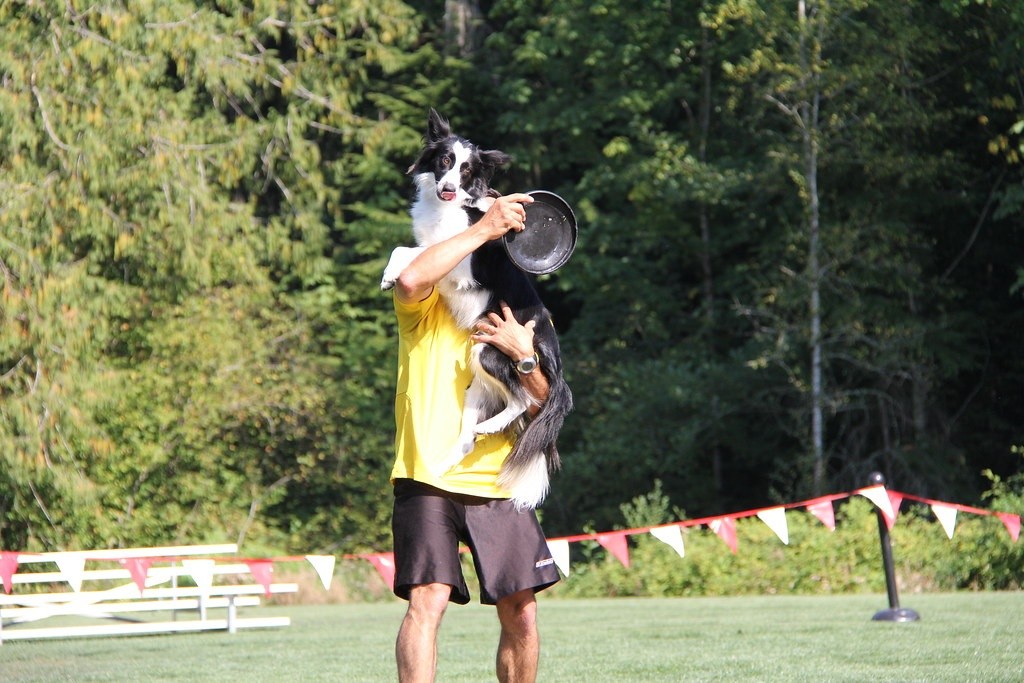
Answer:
[511,354,540,375]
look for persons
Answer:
[391,189,562,683]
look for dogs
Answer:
[404,105,576,513]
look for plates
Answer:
[502,190,578,276]
